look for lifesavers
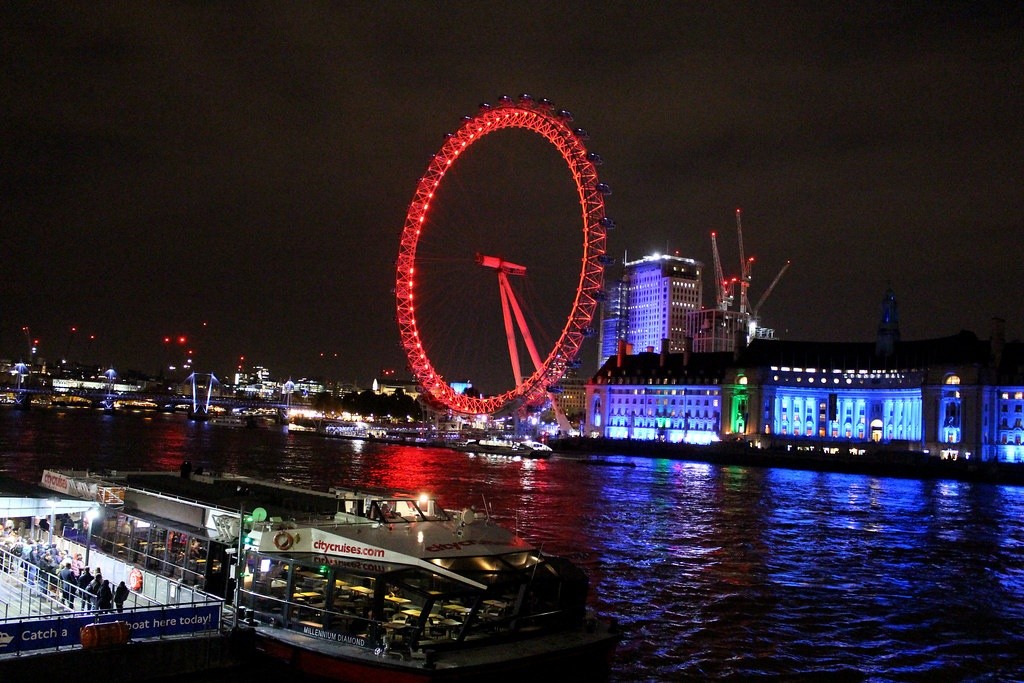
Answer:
[273,532,293,551]
[128,569,143,592]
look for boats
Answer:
[32,462,626,683]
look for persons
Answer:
[365,498,391,518]
[384,584,402,607]
[265,562,324,604]
[179,457,203,486]
[0,515,129,614]
[167,531,201,558]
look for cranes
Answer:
[712,210,790,321]
